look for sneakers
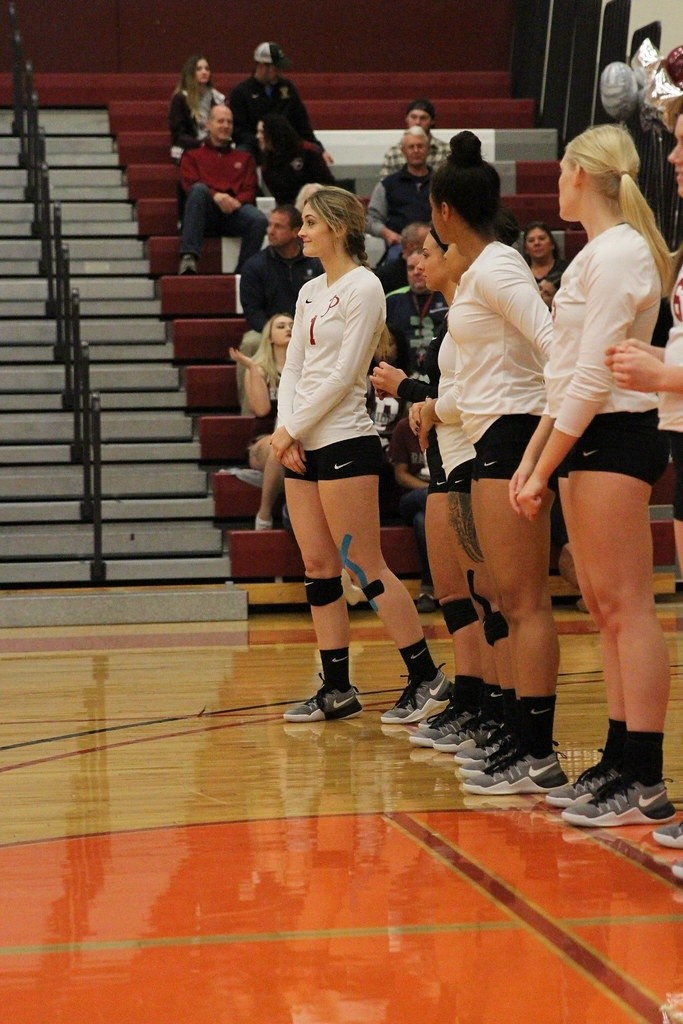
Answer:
[283,673,363,722]
[409,694,570,795]
[416,593,436,612]
[380,662,453,723]
[673,862,683,878]
[179,257,197,275]
[546,748,625,809]
[652,822,683,849]
[561,776,676,827]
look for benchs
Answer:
[107,96,683,593]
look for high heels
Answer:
[255,514,273,531]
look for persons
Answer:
[427,157,555,798]
[507,125,676,827]
[240,197,316,332]
[234,725,683,1024]
[178,103,267,270]
[166,52,226,227]
[269,185,453,725]
[606,95,683,881]
[226,38,683,756]
[228,310,293,531]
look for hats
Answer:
[407,100,436,129]
[255,42,294,66]
